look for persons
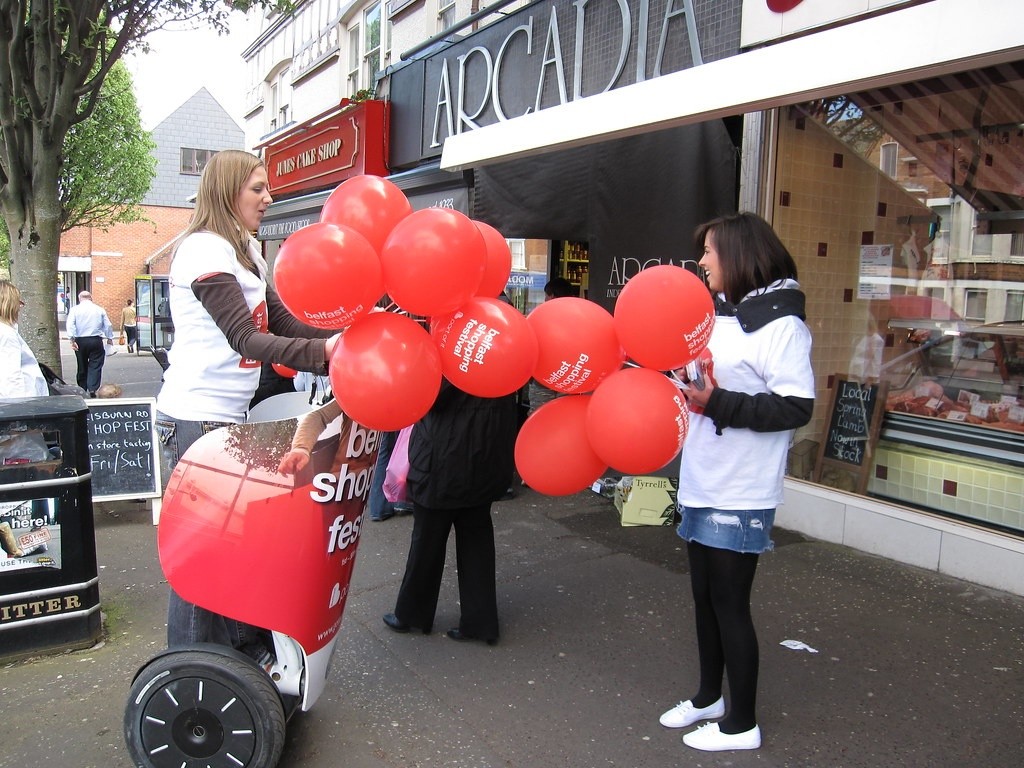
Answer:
[66,291,114,398]
[544,278,572,300]
[0,280,49,399]
[278,400,375,523]
[368,430,413,521]
[66,287,71,315]
[660,213,815,751]
[152,150,342,651]
[381,376,530,644]
[120,300,137,353]
[846,309,885,388]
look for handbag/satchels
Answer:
[119,333,125,345]
[105,343,117,356]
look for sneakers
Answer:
[682,718,761,751]
[660,694,726,728]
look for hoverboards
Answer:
[122,386,383,768]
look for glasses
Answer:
[19,300,25,307]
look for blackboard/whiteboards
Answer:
[818,374,890,472]
[84,397,163,503]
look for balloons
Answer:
[526,297,628,394]
[586,368,690,475]
[431,296,539,398]
[614,265,716,371]
[273,222,383,330]
[328,312,443,431]
[380,207,487,317]
[473,220,512,298]
[514,396,608,496]
[319,174,413,302]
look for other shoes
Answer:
[128,345,134,353]
[90,391,97,398]
[369,512,394,522]
[394,507,414,515]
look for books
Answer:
[685,356,705,391]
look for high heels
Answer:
[383,612,433,636]
[446,626,498,647]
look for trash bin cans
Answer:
[0,396,104,666]
